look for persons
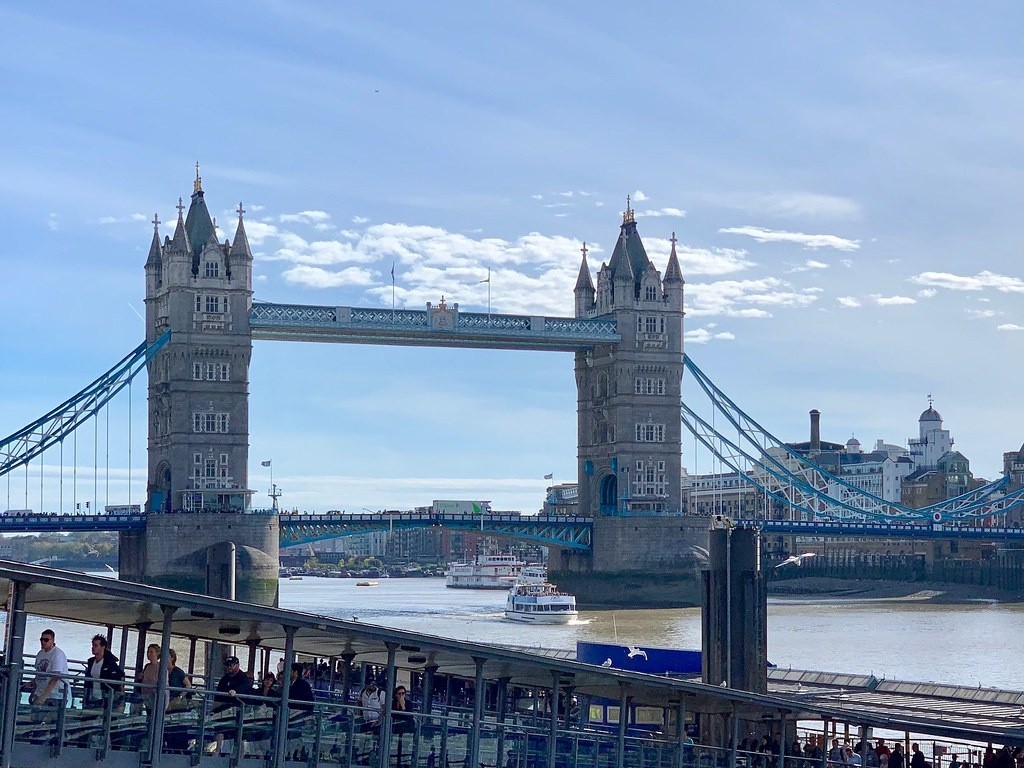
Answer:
[135,643,1024,768]
[20,630,72,744]
[78,632,126,749]
[0,509,712,516]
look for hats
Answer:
[223,656,239,665]
[895,743,902,748]
[291,662,303,671]
[365,674,376,685]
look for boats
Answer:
[441,553,548,589]
[503,582,579,623]
[301,638,775,753]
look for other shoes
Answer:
[120,747,129,751]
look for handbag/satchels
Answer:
[134,679,143,691]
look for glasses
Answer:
[40,637,52,643]
[225,662,235,667]
[397,692,405,696]
[368,683,378,688]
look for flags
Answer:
[261,460,270,467]
